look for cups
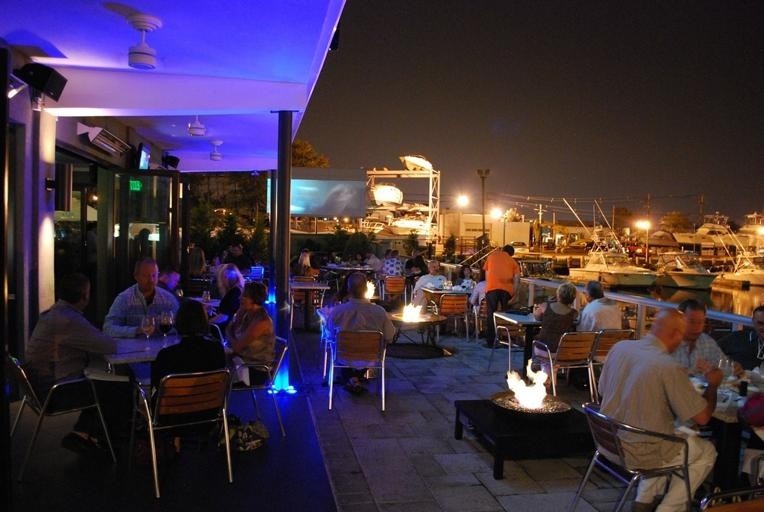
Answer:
[175,288,183,298]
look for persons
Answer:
[569,281,622,391]
[27,216,276,467]
[670,299,744,492]
[297,243,480,393]
[717,306,763,385]
[482,243,521,349]
[598,306,724,512]
[531,282,578,360]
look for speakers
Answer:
[162,155,180,169]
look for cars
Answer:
[584,243,605,251]
[510,241,530,253]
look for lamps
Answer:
[209,139,224,161]
[188,115,206,137]
[77,123,131,156]
[126,13,162,71]
[164,155,180,168]
[45,163,73,212]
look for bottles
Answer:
[202,291,210,303]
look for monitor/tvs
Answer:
[136,142,152,169]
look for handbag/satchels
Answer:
[218,413,270,466]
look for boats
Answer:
[566,214,764,292]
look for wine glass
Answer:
[159,310,174,348]
[142,316,155,351]
[719,360,735,392]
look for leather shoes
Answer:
[633,497,660,512]
[346,382,364,392]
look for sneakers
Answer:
[61,432,100,457]
